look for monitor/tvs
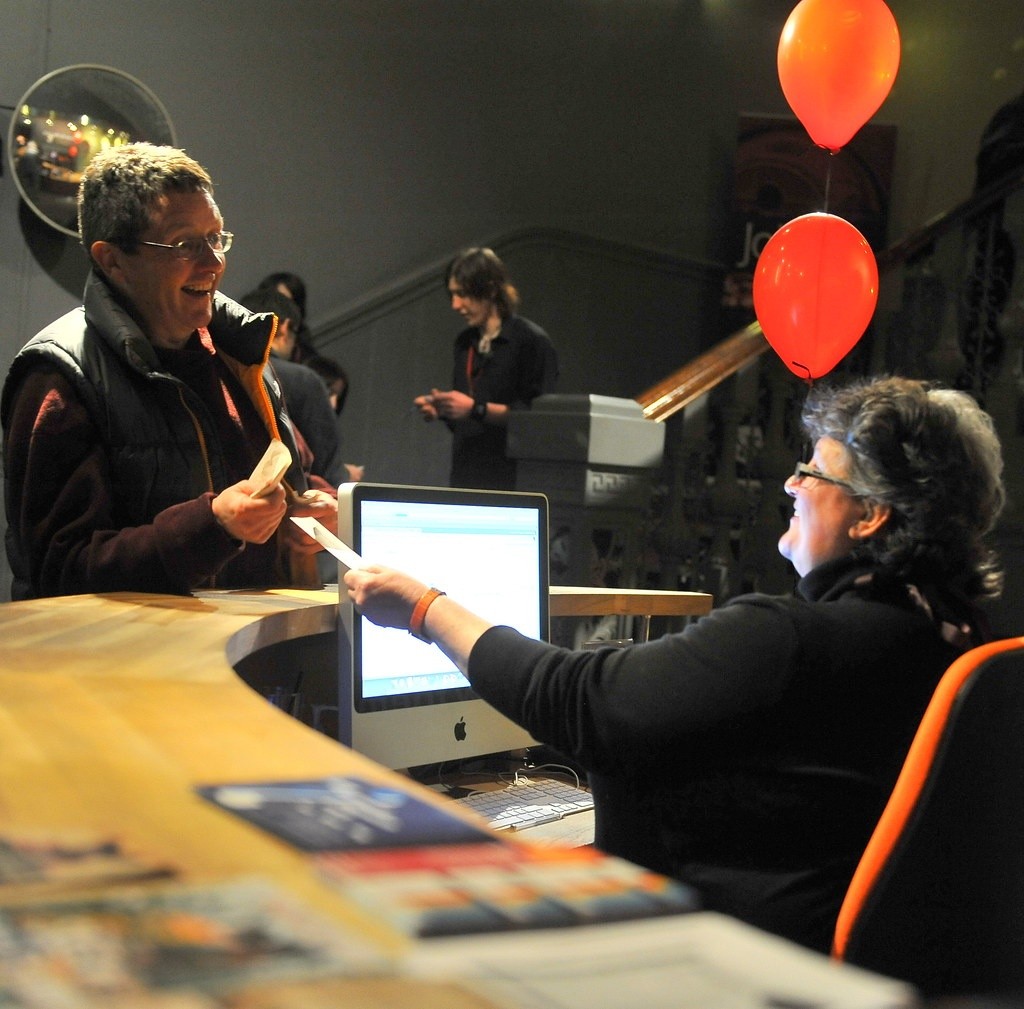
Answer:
[339,482,550,796]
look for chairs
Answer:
[831,635,1024,1009]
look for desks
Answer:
[0,585,923,1009]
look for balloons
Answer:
[752,211,879,379]
[775,0,901,154]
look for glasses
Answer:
[131,231,235,261]
[795,462,870,499]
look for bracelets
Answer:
[408,588,448,646]
[469,397,487,421]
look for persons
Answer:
[238,272,365,488]
[0,143,339,600]
[18,140,50,177]
[413,248,555,491]
[344,378,1005,954]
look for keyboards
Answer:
[453,778,595,832]
